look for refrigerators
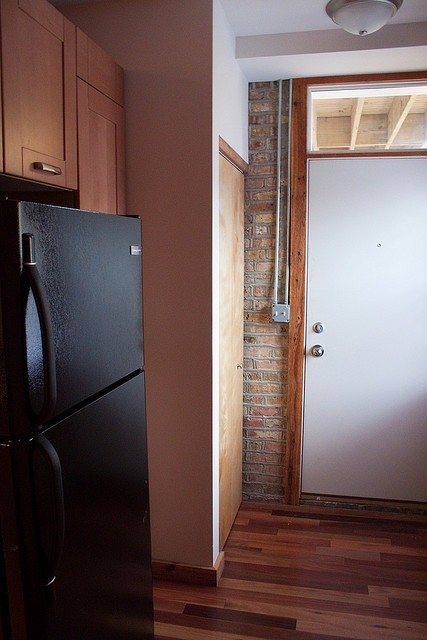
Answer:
[0,201,151,640]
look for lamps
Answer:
[324,0,407,38]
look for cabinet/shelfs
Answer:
[1,2,82,192]
[77,13,128,221]
